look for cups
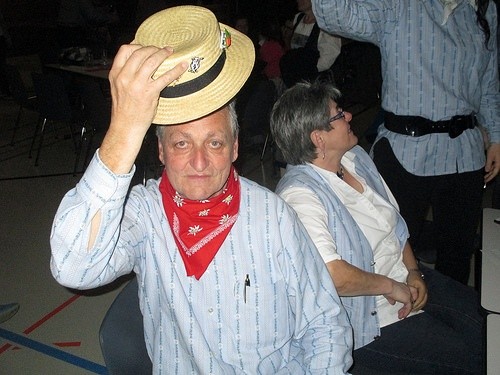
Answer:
[84,49,109,65]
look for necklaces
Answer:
[337,166,344,180]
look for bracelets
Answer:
[409,270,425,280]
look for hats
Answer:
[130,6,255,125]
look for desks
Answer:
[480,207,500,375]
[45,57,114,79]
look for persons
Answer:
[234,0,382,166]
[270,81,486,375]
[50,44,354,375]
[310,0,500,284]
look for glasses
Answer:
[330,105,344,122]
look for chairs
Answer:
[6,73,283,178]
[99,276,153,375]
